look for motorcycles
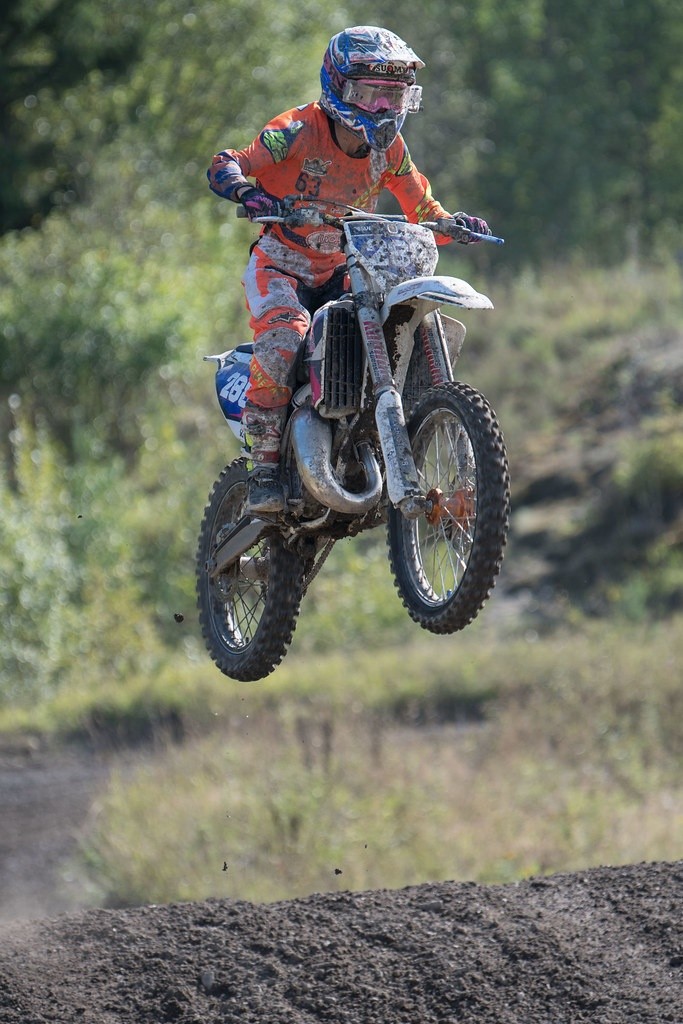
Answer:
[196,194,509,683]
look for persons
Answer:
[207,26,489,512]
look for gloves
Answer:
[452,212,491,242]
[240,188,284,224]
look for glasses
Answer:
[341,79,408,115]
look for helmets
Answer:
[317,26,426,152]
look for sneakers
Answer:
[246,465,285,513]
